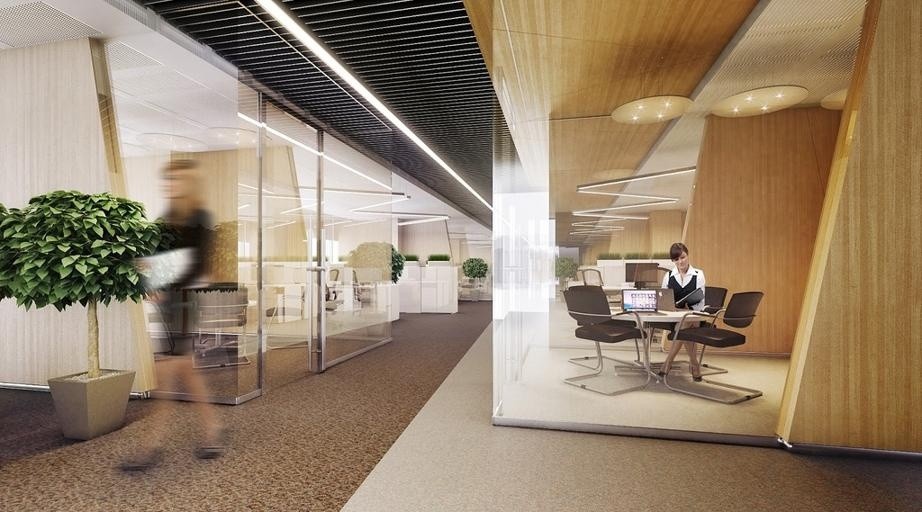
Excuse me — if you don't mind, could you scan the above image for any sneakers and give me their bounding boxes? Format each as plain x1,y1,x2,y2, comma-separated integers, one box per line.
117,421,238,472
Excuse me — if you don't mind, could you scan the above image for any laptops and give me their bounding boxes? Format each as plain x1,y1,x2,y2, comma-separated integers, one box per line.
646,287,690,313
621,288,668,317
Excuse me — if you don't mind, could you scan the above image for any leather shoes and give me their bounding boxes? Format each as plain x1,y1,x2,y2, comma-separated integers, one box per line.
688,365,703,383
658,365,673,378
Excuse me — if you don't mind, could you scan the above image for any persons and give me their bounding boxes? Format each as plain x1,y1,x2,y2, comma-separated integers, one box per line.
657,243,706,382
112,158,222,470
